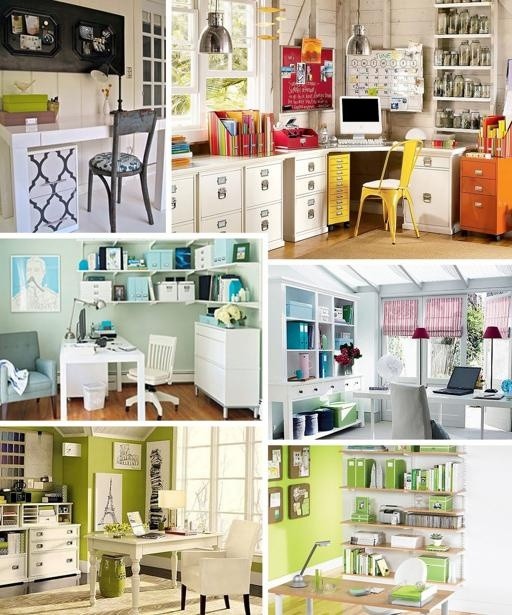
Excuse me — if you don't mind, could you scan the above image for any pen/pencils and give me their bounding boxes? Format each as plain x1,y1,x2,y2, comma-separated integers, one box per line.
484,394,494,397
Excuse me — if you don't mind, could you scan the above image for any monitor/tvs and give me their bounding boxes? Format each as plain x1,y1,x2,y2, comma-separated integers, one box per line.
75,308,90,343
338,94,383,140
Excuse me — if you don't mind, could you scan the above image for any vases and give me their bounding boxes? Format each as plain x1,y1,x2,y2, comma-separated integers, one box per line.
345,365,352,375
103,100,110,117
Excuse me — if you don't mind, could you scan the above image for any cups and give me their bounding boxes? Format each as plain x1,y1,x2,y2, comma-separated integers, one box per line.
329,136,338,148
391,516,398,525
318,128,329,144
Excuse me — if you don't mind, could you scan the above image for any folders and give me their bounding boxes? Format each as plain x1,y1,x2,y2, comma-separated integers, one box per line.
287,321,303,349
303,323,309,349
319,352,328,378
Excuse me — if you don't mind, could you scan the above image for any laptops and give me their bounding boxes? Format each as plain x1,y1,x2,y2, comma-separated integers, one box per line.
433,366,483,396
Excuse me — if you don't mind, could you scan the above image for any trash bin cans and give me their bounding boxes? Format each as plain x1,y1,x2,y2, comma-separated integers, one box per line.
82,382,105,411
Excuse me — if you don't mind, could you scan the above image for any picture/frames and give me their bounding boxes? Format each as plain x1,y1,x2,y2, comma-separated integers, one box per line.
233,242,250,262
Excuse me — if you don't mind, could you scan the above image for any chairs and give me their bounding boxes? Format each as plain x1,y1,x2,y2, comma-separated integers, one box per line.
180,519,262,615
353,148,461,235
87,108,158,233
389,382,432,439
0,330,58,420
158,490,186,532
354,139,425,245
125,334,180,420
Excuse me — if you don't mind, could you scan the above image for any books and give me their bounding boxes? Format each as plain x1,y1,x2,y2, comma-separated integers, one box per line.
403,461,464,492
405,516,464,529
172,137,192,168
345,549,389,575
0,532,25,554
478,119,507,138
220,114,273,152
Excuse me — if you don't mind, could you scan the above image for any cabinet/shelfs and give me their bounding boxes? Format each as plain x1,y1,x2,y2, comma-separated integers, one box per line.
78,240,191,302
433,1,498,133
172,150,351,259
0,524,81,585
459,156,512,241
195,321,261,420
20,501,74,526
269,277,365,440
339,446,464,579
187,239,262,309
0,503,20,526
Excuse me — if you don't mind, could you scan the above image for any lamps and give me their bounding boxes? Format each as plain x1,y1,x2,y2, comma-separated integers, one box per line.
289,540,331,588
62,442,81,457
412,328,430,385
199,0,234,54
64,297,107,339
345,0,370,57
91,60,128,114
483,326,502,393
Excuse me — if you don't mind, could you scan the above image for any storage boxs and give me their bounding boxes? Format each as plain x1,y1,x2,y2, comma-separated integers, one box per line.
286,300,313,320
0,111,56,126
144,250,174,269
154,281,177,302
177,280,195,302
3,95,48,113
320,402,358,428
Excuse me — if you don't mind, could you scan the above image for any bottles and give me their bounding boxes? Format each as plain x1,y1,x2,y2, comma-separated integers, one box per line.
437,8,489,35
435,108,480,129
434,39,491,66
434,72,490,98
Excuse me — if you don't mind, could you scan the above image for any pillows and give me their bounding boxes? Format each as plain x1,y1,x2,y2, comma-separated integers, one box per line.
432,420,451,439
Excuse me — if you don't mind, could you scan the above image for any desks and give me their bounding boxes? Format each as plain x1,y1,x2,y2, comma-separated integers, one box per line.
60,335,145,421
83,533,226,615
353,388,512,439
0,114,166,233
268,575,456,615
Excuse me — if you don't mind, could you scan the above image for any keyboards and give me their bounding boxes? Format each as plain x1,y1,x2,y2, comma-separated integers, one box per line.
339,140,385,147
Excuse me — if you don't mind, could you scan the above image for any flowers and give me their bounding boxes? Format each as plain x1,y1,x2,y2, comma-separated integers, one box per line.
214,304,248,329
102,83,112,100
333,342,362,367
